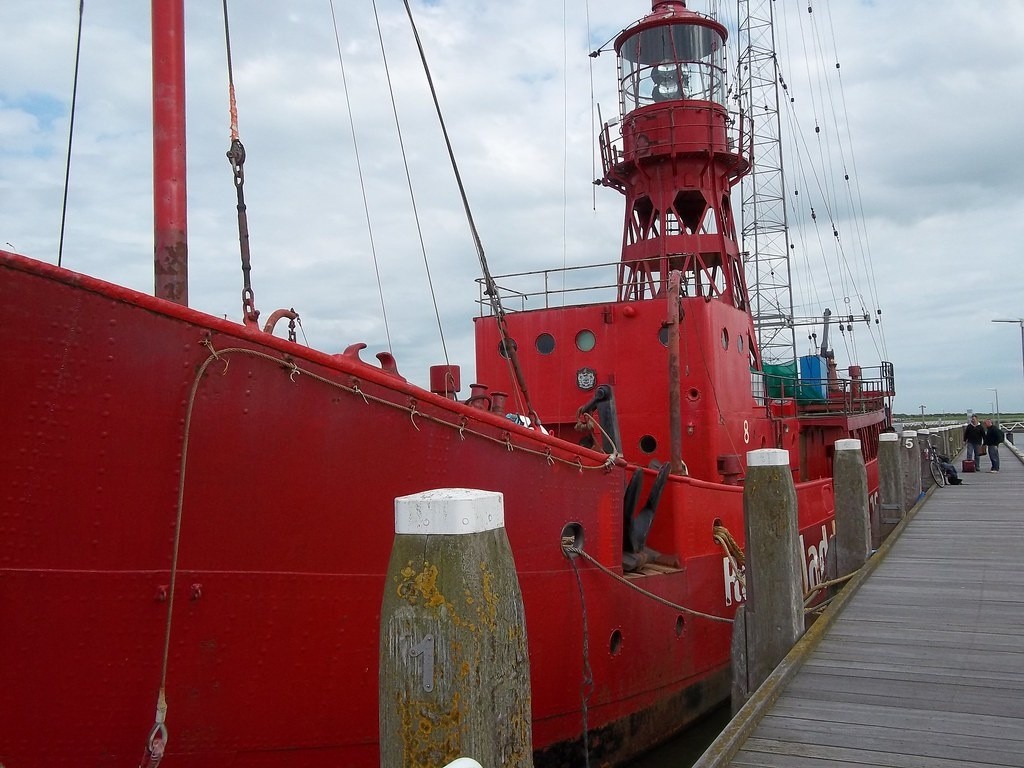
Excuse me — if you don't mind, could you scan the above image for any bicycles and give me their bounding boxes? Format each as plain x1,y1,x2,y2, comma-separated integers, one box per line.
918,433,950,488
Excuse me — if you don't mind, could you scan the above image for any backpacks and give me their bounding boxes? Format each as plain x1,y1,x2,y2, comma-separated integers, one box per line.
993,427,1005,443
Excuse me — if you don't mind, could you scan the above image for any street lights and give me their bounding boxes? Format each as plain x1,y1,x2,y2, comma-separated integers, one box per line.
992,389,1000,428
987,402,994,425
918,404,927,428
991,318,1024,378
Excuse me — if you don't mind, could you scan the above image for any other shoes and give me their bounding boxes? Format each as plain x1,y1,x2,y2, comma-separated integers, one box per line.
977,468,980,471
988,470,999,473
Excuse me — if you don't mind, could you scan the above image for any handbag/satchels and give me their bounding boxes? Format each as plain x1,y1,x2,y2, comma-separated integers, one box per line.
979,446,987,456
962,460,976,472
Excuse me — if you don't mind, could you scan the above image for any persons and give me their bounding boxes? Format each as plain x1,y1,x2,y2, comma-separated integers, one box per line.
983,418,1000,474
964,415,987,471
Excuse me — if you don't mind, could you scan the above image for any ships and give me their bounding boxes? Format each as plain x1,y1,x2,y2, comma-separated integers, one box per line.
1,0,897,767
